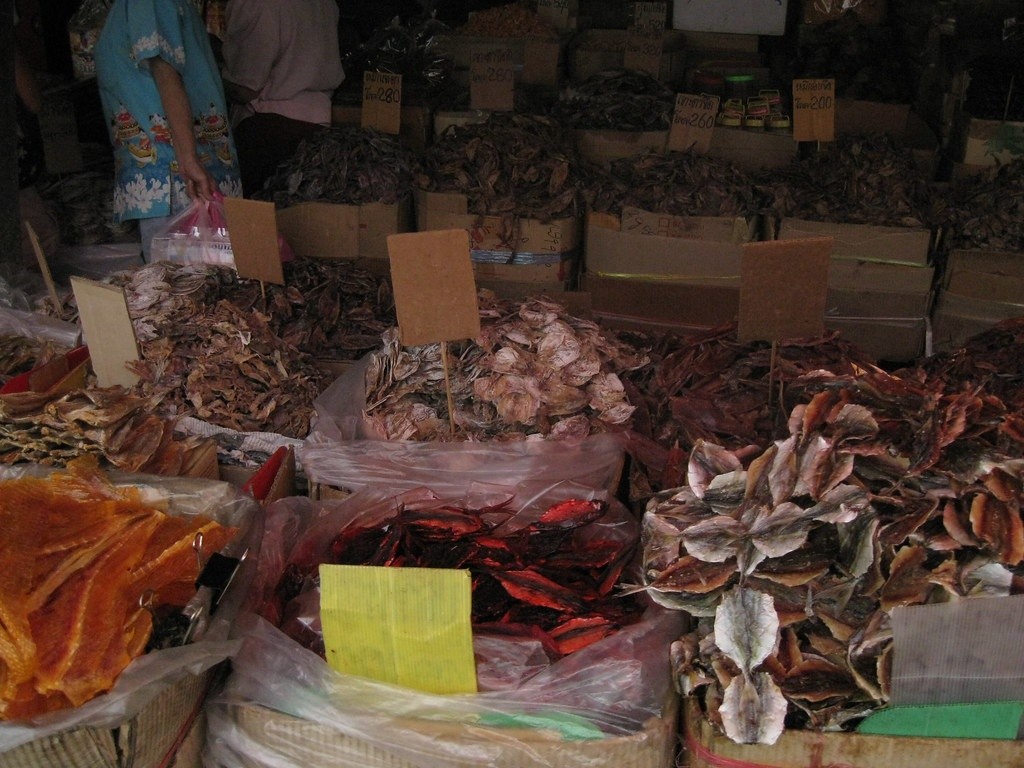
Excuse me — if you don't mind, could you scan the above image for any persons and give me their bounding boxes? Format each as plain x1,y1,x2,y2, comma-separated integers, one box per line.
218,0,345,199
1,0,244,278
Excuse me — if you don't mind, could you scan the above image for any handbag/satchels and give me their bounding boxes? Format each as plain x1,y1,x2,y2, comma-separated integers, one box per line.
151,191,296,270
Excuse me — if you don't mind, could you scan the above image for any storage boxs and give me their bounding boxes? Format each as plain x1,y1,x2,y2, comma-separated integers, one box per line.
1,0,1024,766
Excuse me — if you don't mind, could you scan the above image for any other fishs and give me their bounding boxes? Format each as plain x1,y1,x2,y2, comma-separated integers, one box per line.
0,0,1023,746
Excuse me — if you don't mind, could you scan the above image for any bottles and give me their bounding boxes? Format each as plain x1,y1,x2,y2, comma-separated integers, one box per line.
722,74,755,105
692,71,725,114
